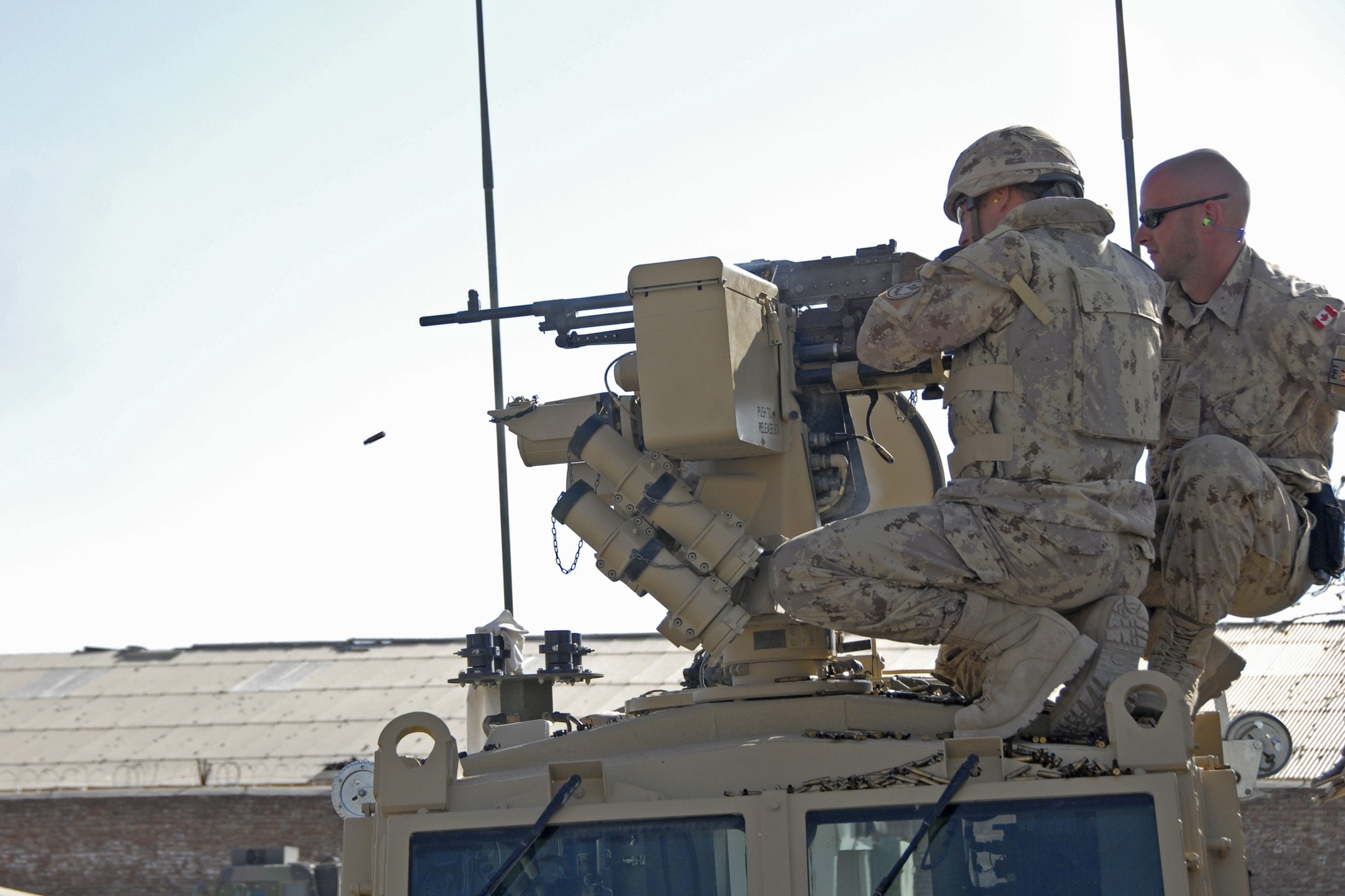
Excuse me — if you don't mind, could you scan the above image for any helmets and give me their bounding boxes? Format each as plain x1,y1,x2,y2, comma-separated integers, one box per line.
943,125,1084,225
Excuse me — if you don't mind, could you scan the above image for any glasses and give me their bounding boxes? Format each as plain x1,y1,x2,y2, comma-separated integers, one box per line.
1139,194,1228,229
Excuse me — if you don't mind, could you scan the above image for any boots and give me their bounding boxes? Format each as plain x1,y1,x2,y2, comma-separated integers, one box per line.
1050,595,1149,740
1134,606,1216,717
940,591,1098,739
1190,634,1247,723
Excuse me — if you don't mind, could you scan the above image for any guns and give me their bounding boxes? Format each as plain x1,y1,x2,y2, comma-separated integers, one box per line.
415,242,971,378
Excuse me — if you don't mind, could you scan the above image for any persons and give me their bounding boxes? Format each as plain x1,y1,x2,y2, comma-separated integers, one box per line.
1132,149,1345,717
767,127,1169,745
